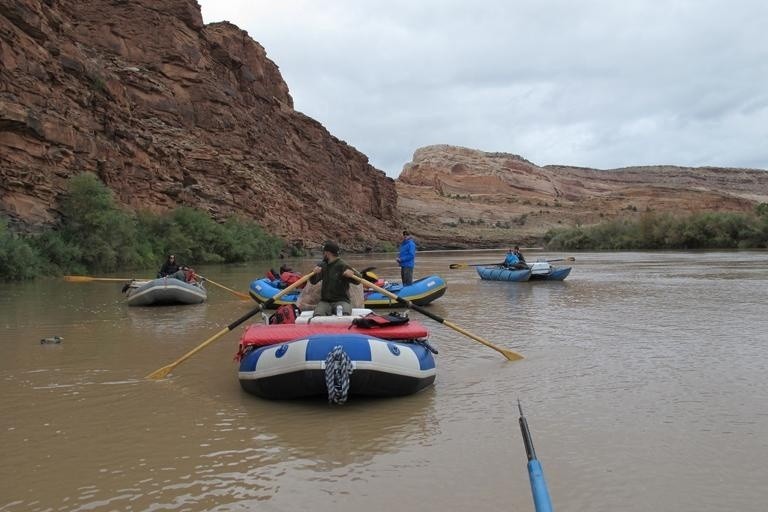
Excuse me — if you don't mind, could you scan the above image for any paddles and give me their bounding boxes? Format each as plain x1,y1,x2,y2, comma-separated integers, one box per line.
449,264,505,269
351,274,524,361
359,266,376,279
192,273,251,301
64,275,150,282
145,266,323,379
527,257,575,263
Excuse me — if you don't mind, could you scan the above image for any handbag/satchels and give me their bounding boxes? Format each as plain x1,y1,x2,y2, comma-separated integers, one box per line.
280,272,306,289
269,304,300,323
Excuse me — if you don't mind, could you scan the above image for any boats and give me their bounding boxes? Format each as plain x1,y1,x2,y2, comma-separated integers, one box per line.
476,265,572,281
248,276,448,307
127,279,207,306
238,333,437,400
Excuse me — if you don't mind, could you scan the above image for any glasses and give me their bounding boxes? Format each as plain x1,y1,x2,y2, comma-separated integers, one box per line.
403,234,410,236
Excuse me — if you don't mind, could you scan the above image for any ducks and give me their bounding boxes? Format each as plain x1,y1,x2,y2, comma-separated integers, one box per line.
40,336,64,344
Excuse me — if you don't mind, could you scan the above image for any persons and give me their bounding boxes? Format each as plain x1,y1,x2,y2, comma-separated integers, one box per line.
505,248,518,266
186,268,195,283
394,230,416,287
503,245,525,268
278,262,307,288
159,254,179,277
308,239,363,317
163,264,189,282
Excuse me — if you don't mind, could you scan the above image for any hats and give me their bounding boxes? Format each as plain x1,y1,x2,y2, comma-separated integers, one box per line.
320,243,338,254
